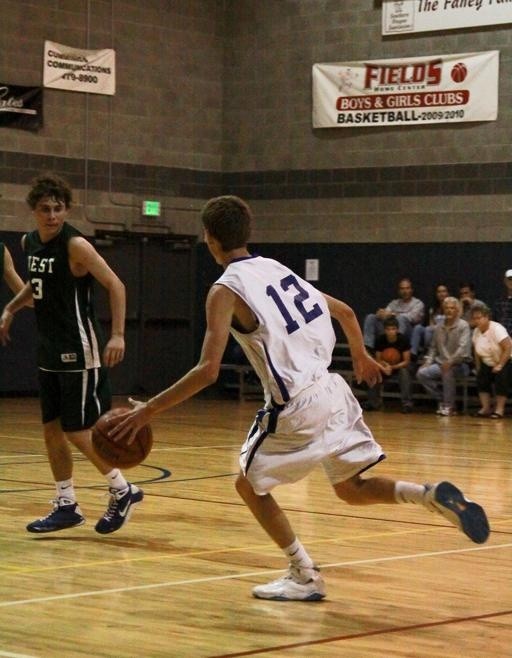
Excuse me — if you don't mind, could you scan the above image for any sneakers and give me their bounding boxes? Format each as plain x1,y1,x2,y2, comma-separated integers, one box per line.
93,480,145,535
421,478,490,544
25,494,85,532
252,568,327,603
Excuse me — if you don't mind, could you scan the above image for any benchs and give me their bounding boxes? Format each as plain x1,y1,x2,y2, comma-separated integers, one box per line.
231,334,512,413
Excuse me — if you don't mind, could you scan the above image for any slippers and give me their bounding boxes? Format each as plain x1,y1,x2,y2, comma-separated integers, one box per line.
470,411,490,418
489,412,505,419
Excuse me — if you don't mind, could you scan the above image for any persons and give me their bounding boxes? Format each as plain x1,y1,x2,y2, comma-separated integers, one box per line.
417,283,454,363
467,306,512,421
360,277,427,364
450,283,495,328
491,266,512,338
106,194,489,605
0,241,38,309
360,318,416,414
413,297,472,417
1,171,145,535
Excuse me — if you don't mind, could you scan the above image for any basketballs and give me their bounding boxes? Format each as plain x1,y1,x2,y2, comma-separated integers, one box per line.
91,407,153,469
382,348,400,365
451,62,468,82
